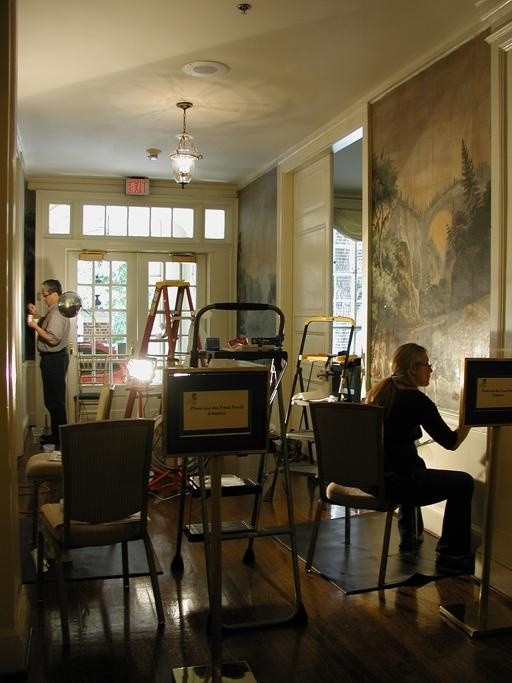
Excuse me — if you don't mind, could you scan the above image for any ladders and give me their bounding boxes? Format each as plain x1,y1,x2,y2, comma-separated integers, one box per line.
170,301,310,639
267,314,354,513
124,279,198,419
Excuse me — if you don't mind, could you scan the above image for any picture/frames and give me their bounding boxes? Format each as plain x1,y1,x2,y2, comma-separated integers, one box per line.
363,35,508,434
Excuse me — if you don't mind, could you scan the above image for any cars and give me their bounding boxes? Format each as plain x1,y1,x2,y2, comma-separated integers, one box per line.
78,342,127,385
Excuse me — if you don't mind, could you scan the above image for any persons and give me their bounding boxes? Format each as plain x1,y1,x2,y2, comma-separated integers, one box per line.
25,279,71,447
363,342,475,573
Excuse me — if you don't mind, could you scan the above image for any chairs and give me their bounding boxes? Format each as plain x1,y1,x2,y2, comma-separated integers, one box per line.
306,399,421,604
25,384,115,551
25,417,167,653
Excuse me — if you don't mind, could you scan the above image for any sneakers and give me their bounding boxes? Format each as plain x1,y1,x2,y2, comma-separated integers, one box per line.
397,535,423,550
434,550,475,562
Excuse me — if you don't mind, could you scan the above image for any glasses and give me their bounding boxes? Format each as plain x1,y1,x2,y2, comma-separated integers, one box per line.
416,360,431,367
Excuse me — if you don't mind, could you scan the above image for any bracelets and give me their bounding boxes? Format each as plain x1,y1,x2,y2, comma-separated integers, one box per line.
35,327,38,331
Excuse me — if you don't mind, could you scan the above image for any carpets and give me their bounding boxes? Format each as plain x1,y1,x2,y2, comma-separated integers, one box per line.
262,509,443,594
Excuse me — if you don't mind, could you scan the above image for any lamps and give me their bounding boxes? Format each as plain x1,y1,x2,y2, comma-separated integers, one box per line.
57,290,111,353
169,102,204,191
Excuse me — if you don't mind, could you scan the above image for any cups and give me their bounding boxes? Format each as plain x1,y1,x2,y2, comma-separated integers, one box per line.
28,315,34,322
43,444,55,460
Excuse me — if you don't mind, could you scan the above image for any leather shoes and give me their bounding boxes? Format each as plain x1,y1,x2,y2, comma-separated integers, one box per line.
41,433,55,438
40,437,58,443
